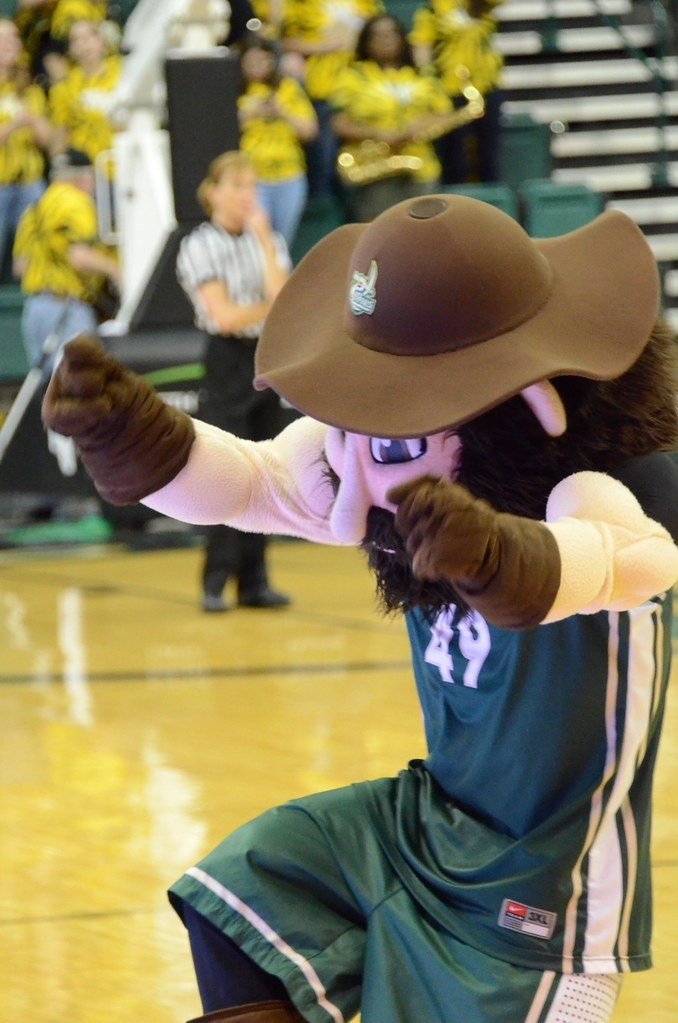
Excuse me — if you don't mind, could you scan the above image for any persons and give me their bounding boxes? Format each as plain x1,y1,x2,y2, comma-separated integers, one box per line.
0,0,128,480
175,153,292,612
234,0,505,248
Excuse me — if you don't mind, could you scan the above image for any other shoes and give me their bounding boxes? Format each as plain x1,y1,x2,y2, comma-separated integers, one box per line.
203,588,226,611
238,586,288,607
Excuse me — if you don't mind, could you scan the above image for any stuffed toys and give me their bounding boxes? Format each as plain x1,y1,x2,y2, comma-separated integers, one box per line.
42,192,678,1023
